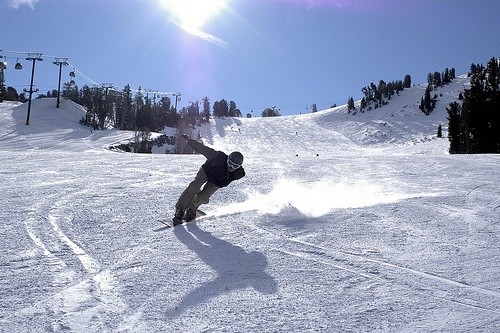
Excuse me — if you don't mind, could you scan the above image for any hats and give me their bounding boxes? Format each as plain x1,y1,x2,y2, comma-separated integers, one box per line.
229,152,244,165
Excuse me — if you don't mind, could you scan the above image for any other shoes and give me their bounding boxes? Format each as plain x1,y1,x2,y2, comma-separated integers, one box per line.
186,208,196,219
176,208,185,219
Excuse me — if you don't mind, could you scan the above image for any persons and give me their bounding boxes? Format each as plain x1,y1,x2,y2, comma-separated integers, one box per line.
172,134,246,226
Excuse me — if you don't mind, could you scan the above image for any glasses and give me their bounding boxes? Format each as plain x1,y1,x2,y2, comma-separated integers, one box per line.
227,159,240,169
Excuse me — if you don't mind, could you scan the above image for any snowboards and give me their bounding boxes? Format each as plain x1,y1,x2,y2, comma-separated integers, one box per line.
157,208,207,228
